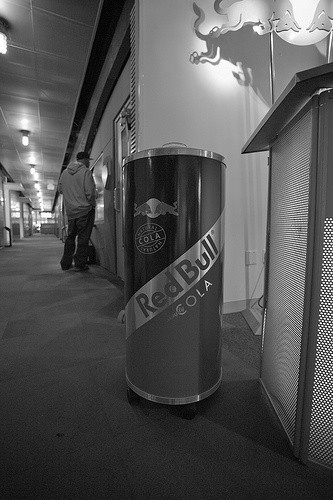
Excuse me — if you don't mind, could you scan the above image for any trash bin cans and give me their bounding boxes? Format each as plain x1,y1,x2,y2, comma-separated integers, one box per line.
121,142,227,407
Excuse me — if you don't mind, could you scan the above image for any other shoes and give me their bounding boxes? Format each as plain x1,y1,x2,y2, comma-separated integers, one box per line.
73,264,89,271
62,264,73,270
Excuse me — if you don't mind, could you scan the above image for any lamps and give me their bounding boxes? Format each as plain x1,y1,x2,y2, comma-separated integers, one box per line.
0,17,10,54
19,129,31,147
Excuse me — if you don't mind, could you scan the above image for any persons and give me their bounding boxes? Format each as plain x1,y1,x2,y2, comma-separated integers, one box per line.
58,152,96,272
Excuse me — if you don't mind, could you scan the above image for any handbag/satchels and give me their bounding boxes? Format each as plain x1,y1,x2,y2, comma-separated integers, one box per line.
86,239,96,264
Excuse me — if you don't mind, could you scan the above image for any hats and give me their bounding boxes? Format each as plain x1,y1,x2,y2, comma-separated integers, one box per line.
77,152,94,160
